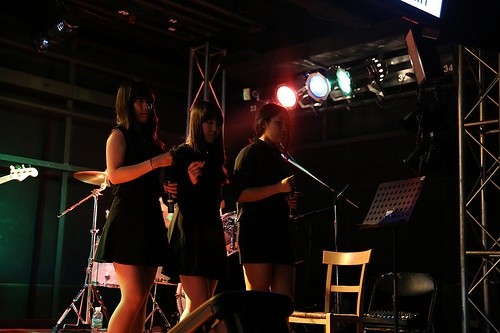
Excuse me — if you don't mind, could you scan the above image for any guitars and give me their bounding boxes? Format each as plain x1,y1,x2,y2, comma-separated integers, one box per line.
0,163,40,186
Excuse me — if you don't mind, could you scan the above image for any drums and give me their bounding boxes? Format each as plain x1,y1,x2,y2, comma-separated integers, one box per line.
155,267,178,285
90,235,120,288
219,212,241,254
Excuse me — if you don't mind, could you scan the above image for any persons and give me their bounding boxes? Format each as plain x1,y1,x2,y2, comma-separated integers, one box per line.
94,81,197,333
158,100,303,333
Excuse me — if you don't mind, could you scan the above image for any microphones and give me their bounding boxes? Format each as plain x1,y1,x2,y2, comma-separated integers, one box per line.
288,180,297,219
167,176,175,212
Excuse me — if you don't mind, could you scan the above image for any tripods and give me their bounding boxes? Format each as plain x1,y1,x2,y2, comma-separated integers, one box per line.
50,178,110,333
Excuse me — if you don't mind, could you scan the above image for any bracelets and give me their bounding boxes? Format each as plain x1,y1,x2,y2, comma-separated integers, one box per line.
149,158,155,169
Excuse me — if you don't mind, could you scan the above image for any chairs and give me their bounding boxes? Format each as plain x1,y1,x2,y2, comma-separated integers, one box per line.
287,249,372,333
363,272,437,333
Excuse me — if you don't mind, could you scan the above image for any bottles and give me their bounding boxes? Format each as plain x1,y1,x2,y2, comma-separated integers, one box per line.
90,307,103,333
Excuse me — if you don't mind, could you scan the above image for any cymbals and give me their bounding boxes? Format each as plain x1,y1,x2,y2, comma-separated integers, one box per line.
73,171,111,187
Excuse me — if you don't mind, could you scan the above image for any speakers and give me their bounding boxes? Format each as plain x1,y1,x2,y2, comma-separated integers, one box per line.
405,25,480,94
168,291,294,333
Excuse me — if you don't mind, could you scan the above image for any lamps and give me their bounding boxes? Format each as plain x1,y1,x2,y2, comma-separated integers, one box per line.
34,15,81,54
277,58,389,110
